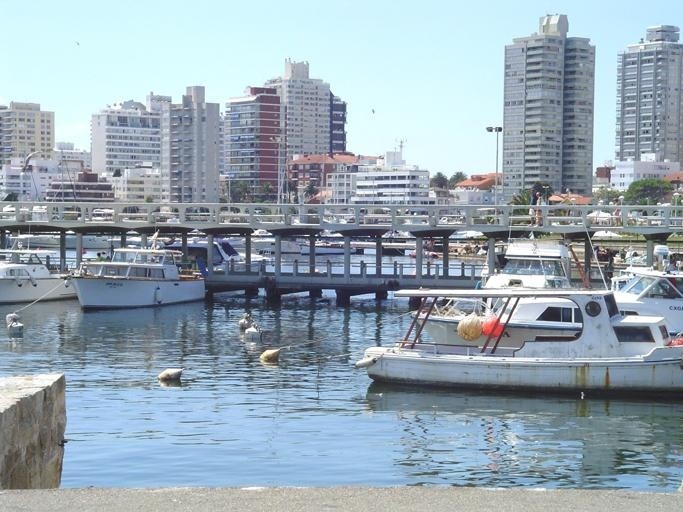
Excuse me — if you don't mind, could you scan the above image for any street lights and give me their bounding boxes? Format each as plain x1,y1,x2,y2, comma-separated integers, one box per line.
564,186,571,224
672,192,681,226
543,185,550,205
486,127,504,223
619,195,625,224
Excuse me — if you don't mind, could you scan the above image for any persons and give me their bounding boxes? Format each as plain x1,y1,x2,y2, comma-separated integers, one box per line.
612,198,622,227
533,191,543,226
528,192,535,227
